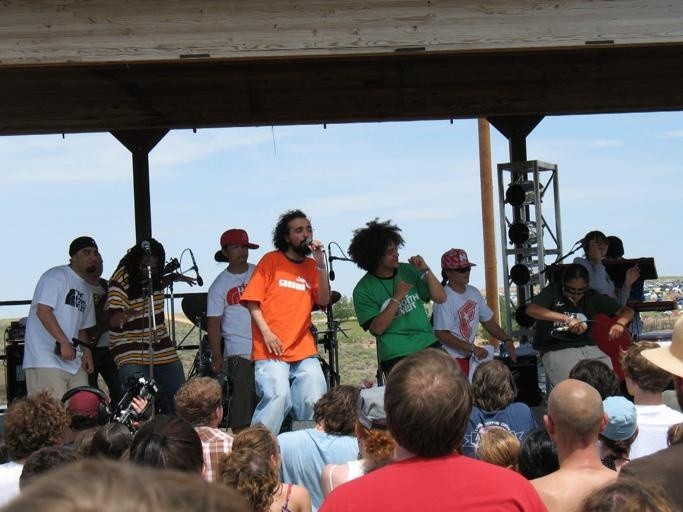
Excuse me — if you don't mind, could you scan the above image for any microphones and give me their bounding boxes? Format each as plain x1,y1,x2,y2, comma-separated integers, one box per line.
556,321,587,332
190,252,203,286
328,246,335,281
141,240,152,262
305,239,325,253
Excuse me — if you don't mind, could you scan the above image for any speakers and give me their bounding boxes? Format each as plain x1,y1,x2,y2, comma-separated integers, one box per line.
493,355,541,407
5,344,27,408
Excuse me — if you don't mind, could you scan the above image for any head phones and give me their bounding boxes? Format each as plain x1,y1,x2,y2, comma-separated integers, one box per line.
60,386,112,425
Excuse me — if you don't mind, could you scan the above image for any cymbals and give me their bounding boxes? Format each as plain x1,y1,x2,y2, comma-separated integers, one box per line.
313,291,341,310
181,293,209,332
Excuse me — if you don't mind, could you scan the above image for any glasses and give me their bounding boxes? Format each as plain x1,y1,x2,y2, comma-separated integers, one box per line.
452,267,471,273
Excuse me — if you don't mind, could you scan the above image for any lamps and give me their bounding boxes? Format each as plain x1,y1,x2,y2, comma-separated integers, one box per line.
496,159,562,352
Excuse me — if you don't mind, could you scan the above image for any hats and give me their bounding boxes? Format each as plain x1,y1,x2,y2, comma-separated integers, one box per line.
67,388,104,418
69,236,97,257
597,395,638,442
441,249,475,271
640,311,683,380
220,228,259,249
355,386,395,431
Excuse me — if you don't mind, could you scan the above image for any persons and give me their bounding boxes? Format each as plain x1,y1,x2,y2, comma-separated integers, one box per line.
0,314,683,511
239,209,332,434
434,231,645,399
23,229,259,432
347,214,453,380
